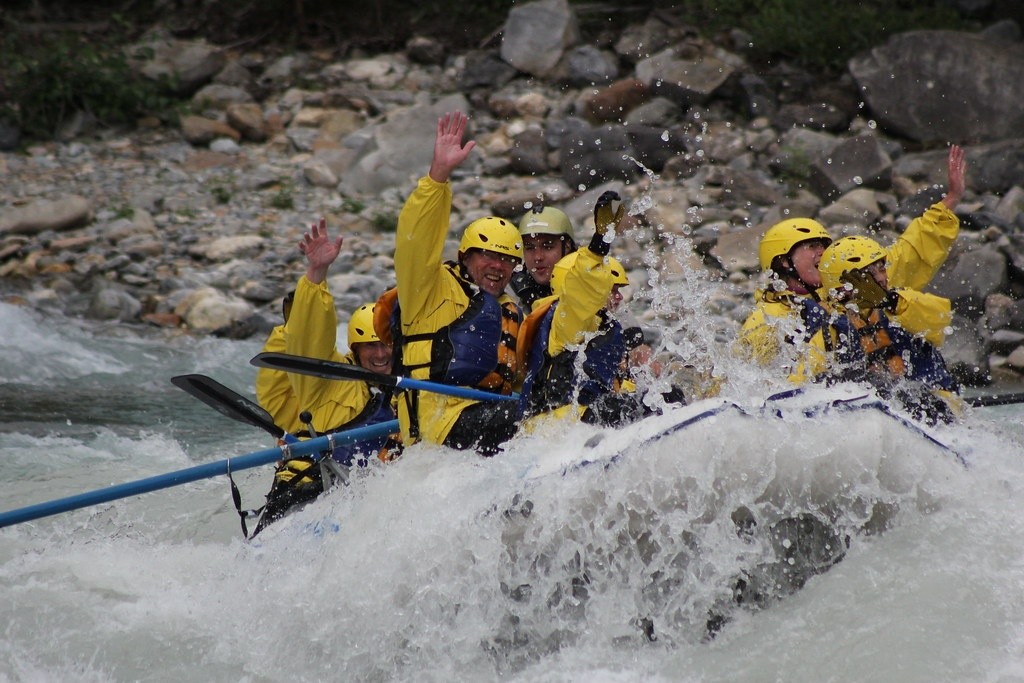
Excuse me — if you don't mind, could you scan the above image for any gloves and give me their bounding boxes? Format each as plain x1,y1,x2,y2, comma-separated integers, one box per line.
593,191,626,235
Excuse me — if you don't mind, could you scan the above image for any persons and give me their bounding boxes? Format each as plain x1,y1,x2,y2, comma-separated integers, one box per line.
528,190,628,420
255,289,326,532
505,199,578,415
393,117,526,458
583,326,685,429
285,217,393,481
705,144,968,421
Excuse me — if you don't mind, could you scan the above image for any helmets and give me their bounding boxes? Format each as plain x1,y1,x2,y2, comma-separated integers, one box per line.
550,251,630,295
518,206,576,251
459,216,523,265
759,217,833,273
347,303,379,348
818,236,888,292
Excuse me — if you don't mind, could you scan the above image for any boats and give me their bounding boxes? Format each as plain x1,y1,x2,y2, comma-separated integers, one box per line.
249,389,970,545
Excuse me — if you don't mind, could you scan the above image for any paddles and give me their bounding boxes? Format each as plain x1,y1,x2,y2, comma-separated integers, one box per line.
248,350,524,405
169,373,355,465
0,417,401,529
